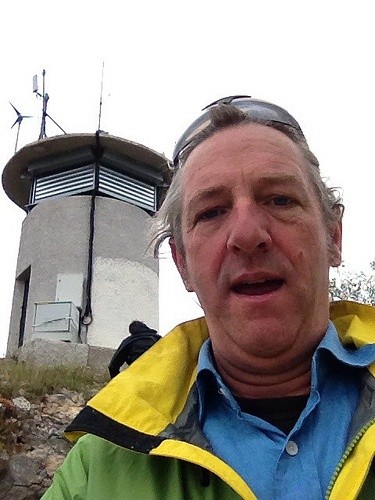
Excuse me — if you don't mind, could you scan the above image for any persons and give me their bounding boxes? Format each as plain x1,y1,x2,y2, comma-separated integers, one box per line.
40,99,375,500
108,321,163,380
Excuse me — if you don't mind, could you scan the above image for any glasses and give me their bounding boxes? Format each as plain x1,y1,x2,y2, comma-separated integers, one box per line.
172,93,298,165
